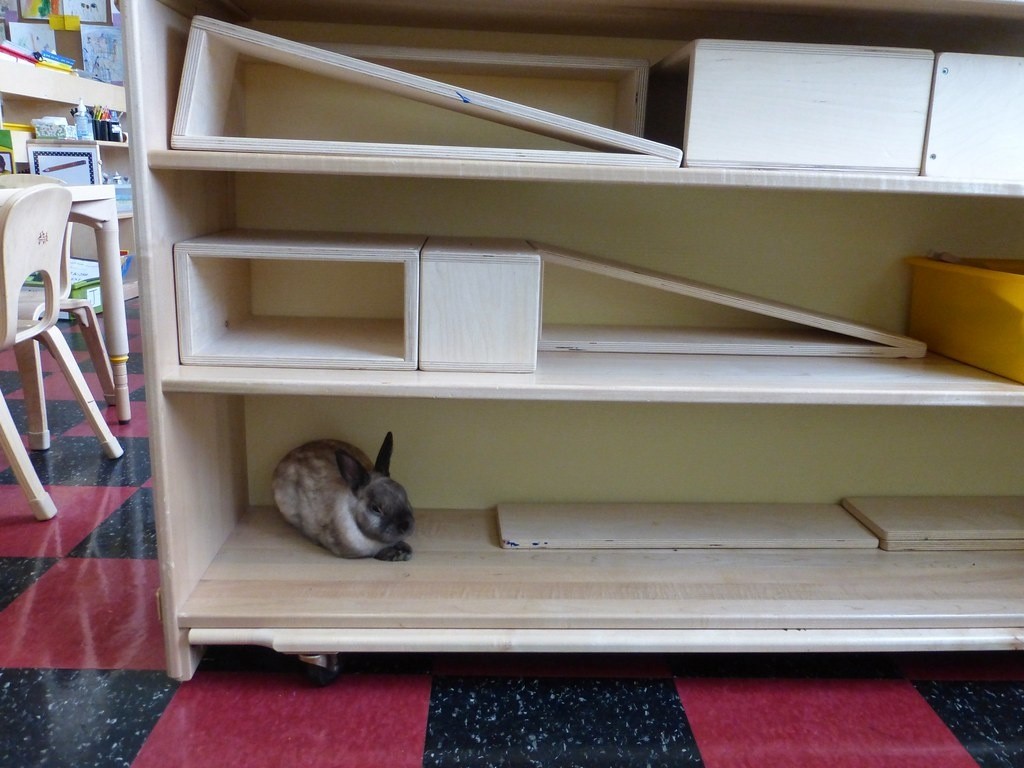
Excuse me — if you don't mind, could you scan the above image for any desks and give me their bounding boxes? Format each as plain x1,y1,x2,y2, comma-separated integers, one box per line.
0,183,132,424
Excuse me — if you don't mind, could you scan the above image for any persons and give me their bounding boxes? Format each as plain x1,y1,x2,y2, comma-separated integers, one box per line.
0,153,11,176
113,0,121,27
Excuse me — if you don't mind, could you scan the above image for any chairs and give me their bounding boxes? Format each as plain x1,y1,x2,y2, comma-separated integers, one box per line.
0,171,115,451
0,183,125,520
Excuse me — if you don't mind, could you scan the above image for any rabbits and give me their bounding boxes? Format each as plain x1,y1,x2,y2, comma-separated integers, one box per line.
267,431,415,562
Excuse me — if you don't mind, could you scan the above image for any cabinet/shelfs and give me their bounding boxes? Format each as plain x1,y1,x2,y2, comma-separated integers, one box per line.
119,1,1023,681
0,52,138,301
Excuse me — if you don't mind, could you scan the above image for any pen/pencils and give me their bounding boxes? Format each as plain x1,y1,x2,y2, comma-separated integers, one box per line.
69,104,124,122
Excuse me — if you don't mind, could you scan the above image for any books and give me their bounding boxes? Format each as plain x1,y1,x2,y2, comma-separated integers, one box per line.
0,38,76,74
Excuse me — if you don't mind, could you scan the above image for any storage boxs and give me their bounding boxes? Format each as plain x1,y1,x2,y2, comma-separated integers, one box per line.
908,256,1024,383
120,254,138,284
21,272,104,320
115,184,134,214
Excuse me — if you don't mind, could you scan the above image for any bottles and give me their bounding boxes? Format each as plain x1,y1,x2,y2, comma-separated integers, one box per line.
74,97,94,141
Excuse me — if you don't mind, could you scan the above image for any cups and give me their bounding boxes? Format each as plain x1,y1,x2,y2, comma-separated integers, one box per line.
91,117,124,142
